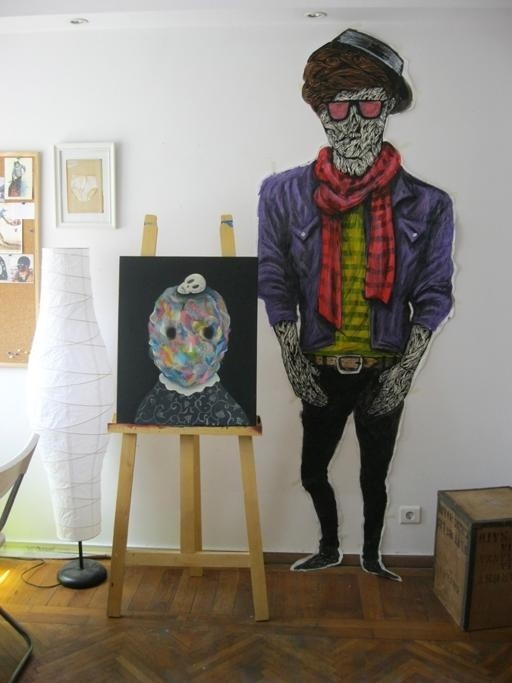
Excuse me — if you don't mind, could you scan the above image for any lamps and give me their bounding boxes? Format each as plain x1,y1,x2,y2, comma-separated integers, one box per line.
28,247,113,590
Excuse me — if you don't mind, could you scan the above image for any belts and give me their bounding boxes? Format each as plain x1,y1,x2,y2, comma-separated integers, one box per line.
301,350,404,376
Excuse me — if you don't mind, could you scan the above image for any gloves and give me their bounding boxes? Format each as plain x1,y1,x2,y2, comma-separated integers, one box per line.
272,320,329,409
366,323,432,418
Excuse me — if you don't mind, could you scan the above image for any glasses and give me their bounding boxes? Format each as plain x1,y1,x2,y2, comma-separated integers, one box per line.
320,95,395,122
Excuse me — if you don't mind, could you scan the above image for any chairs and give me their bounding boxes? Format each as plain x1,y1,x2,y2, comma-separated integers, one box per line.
0,433,39,683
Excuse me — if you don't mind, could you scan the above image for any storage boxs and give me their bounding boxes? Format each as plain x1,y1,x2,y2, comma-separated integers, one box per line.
430,485,512,633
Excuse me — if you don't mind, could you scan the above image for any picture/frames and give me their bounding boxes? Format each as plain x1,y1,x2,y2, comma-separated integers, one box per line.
0,151,42,368
52,141,115,231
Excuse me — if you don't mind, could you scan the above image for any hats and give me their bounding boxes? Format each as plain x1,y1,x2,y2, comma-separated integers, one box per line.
305,27,410,102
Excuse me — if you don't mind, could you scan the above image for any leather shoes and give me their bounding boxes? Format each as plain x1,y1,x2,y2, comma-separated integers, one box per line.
294,549,340,569
363,559,398,580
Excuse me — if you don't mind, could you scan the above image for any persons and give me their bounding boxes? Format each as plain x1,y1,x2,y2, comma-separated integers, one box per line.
13,256,34,283
257,26,454,584
